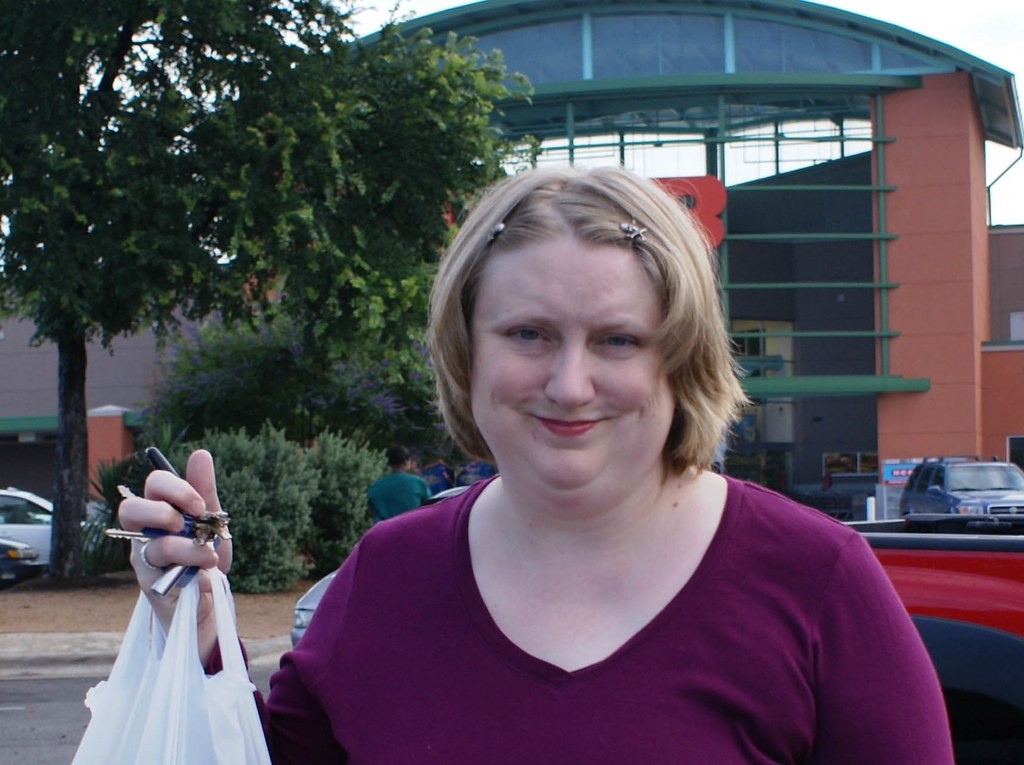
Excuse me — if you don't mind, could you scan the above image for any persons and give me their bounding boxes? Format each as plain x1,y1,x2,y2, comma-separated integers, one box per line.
364,445,431,523
119,164,954,765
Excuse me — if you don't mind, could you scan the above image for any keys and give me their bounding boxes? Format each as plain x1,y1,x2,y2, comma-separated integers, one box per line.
104,446,233,596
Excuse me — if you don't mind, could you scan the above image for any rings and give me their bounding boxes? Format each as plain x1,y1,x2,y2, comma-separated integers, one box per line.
140,542,158,571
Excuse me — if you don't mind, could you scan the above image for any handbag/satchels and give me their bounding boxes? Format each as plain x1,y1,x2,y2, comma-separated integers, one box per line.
71,562,271,765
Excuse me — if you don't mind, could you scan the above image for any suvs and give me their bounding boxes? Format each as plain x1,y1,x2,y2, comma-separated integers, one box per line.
899,455,1024,515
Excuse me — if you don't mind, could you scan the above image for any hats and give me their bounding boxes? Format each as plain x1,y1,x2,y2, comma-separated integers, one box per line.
385,443,412,465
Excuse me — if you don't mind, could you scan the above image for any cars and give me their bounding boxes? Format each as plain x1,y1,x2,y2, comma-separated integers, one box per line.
0,486,112,588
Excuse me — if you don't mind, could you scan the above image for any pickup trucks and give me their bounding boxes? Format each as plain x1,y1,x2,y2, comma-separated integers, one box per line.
811,515,1024,765
